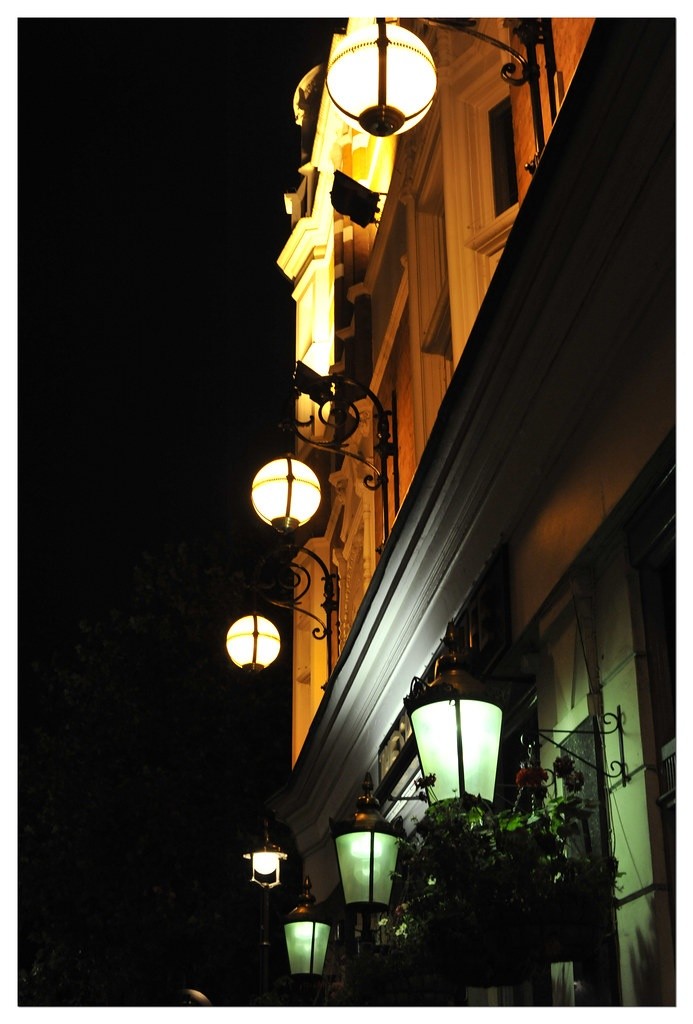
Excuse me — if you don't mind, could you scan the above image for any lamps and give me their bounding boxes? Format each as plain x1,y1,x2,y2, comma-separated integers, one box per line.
281,876,334,975
330,772,401,911
226,18,552,674
400,622,628,809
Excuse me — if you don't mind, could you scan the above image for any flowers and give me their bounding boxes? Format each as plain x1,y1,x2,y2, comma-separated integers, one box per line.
322,753,607,1007
